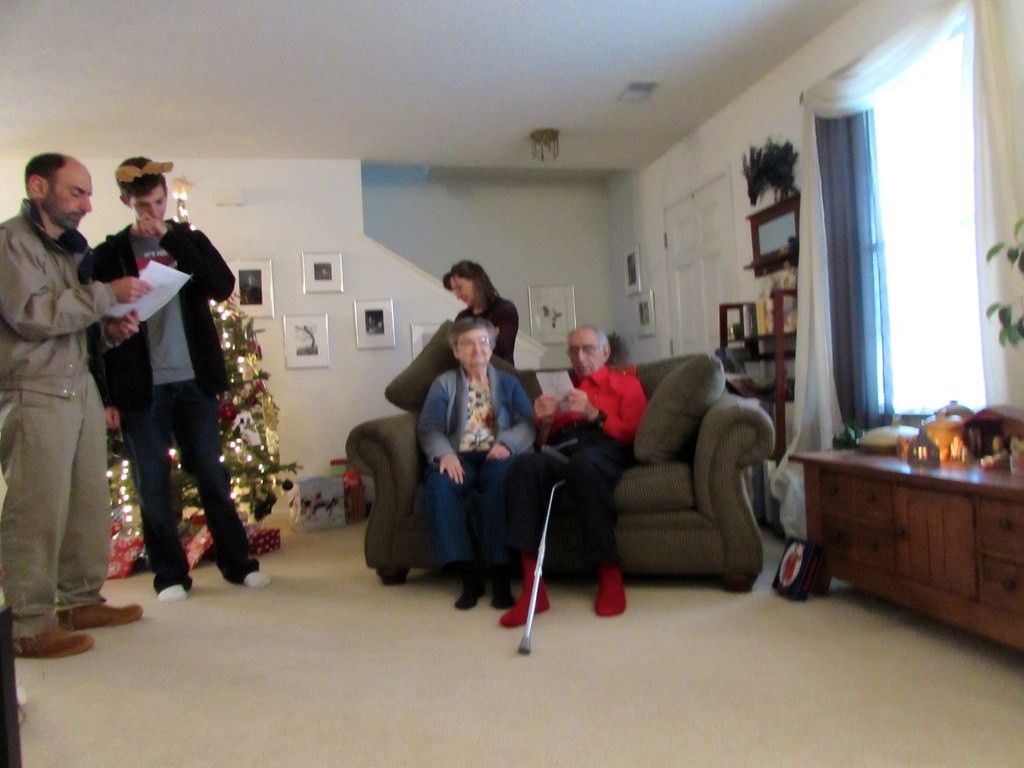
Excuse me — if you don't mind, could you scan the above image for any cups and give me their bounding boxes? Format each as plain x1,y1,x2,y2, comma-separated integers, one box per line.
742,302,758,338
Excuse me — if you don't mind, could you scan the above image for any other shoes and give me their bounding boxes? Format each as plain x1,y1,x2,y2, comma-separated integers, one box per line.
58,605,143,627
13,632,92,657
455,577,486,609
491,575,517,609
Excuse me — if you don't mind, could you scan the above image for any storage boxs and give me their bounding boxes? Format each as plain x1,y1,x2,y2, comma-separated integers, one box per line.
288,457,374,531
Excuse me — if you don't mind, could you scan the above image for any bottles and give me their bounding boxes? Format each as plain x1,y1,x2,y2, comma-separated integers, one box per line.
992,433,1024,476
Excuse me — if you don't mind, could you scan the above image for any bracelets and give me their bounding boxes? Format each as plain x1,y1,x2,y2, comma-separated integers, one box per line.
594,410,606,428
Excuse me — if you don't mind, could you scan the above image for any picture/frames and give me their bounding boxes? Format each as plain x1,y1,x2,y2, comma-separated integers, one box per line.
621,245,642,298
302,252,344,292
528,285,577,346
231,260,275,319
354,298,395,349
636,289,658,336
411,323,443,363
283,312,329,370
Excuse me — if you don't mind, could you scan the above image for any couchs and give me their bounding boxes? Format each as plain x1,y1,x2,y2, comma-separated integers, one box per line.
345,352,775,592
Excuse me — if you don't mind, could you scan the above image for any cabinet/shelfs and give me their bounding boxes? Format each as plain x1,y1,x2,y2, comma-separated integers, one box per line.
790,450,1024,650
719,289,796,460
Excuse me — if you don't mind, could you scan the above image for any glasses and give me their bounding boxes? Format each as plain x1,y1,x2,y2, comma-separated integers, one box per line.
456,336,491,349
568,346,602,354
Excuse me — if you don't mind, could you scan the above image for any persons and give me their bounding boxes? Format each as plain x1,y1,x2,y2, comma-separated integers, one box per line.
443,261,519,367
86,157,269,601
417,319,537,609
0,154,154,659
241,275,261,304
500,326,647,627
781,543,801,583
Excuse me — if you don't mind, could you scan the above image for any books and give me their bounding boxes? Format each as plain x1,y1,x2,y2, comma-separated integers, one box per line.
772,538,823,600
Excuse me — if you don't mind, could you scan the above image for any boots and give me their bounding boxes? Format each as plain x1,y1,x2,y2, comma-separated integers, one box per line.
500,559,550,627
596,569,626,615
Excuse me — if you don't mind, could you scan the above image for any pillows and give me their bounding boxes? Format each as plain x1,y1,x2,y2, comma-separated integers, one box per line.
633,352,725,461
383,319,515,412
